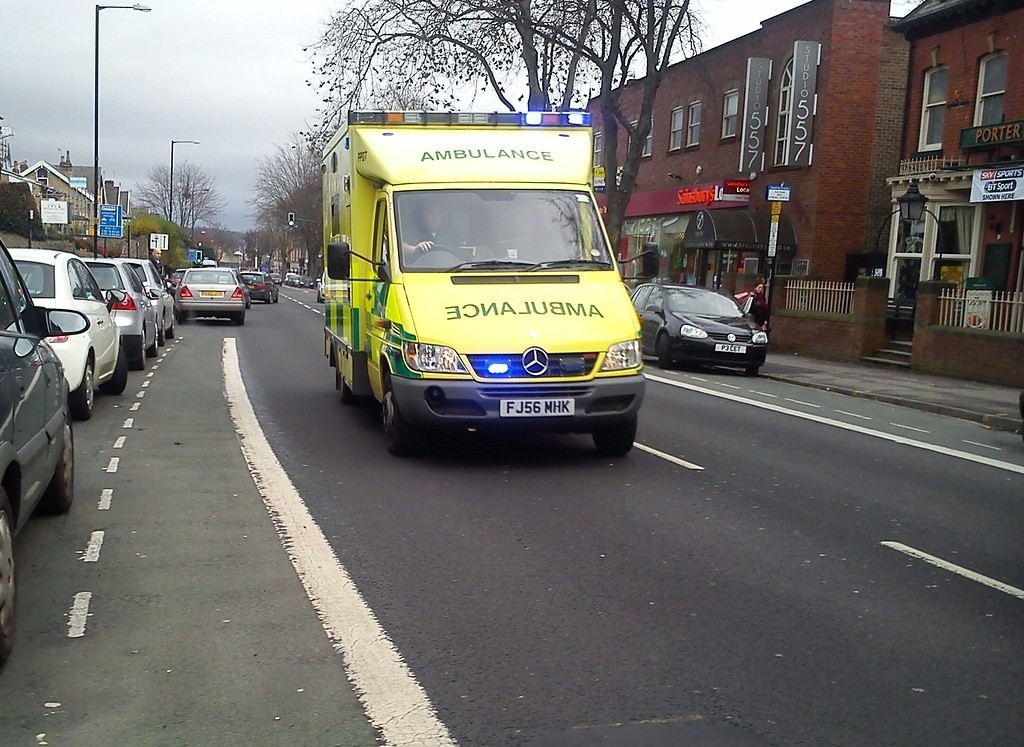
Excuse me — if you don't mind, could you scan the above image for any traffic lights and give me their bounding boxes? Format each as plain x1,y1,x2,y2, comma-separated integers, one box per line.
195,250,203,265
288,212,295,226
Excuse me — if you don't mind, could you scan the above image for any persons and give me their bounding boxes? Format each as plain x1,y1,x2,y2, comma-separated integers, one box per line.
148,248,171,288
401,198,476,264
743,280,771,331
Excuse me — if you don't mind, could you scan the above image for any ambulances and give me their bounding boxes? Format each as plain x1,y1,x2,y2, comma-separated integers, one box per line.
321,107,647,458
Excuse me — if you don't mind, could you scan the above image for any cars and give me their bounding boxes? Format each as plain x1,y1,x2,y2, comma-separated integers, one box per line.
7,246,130,419
67,256,163,370
0,237,75,665
221,267,252,309
94,256,178,346
270,271,314,288
629,280,770,376
315,271,326,303
175,267,247,325
238,270,280,304
166,269,187,298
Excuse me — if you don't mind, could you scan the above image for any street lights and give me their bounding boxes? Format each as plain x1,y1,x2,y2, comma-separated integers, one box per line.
93,4,153,261
192,189,210,242
169,140,200,221
